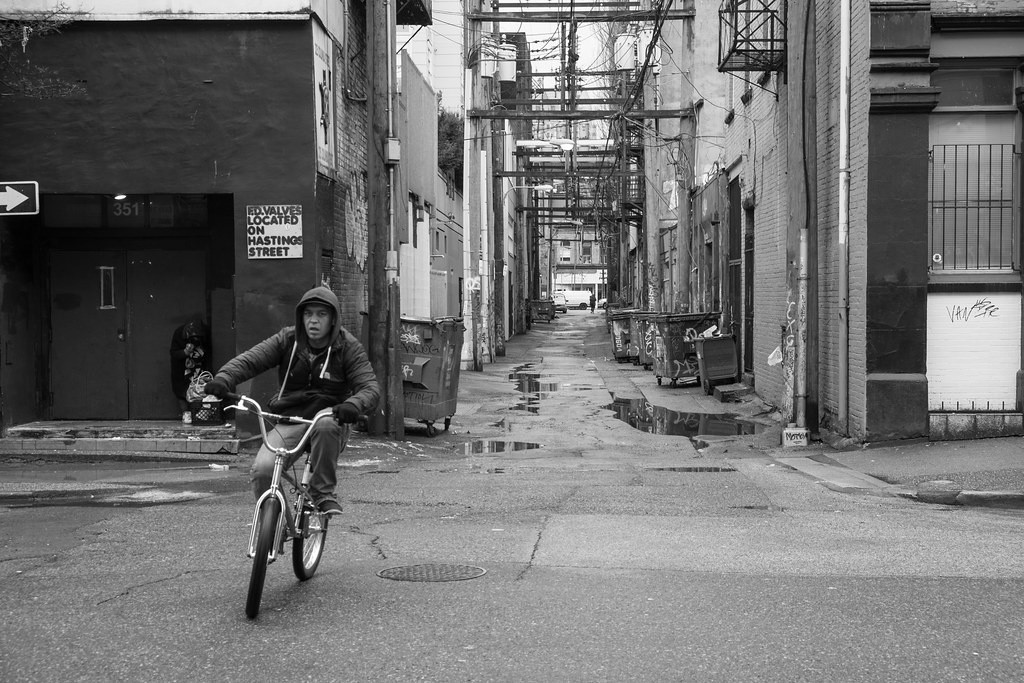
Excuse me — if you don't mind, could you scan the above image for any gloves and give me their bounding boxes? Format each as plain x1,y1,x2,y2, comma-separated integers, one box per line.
205,376,229,399
332,400,359,426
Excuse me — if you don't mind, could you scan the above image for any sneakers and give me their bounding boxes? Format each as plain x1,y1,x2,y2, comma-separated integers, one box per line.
182,411,192,424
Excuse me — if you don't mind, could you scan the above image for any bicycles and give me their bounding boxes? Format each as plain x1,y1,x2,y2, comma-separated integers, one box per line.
222,390,369,619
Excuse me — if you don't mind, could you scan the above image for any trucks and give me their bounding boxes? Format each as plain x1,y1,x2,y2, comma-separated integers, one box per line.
549,292,569,313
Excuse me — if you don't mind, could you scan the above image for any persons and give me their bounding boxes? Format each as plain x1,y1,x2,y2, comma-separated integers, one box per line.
170,318,211,423
590,294,596,314
204,287,380,515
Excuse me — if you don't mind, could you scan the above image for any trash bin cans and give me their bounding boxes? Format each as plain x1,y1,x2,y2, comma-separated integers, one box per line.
607,307,738,395
357,309,467,436
530,300,554,323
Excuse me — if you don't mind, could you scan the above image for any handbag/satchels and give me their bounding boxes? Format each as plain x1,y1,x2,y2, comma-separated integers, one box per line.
185,371,215,403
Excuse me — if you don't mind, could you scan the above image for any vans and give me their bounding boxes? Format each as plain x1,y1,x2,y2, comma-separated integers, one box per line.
555,290,592,310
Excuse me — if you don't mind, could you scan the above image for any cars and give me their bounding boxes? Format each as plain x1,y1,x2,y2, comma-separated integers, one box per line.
597,298,607,309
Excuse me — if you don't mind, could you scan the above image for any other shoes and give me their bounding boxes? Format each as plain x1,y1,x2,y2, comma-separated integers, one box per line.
318,503,343,515
